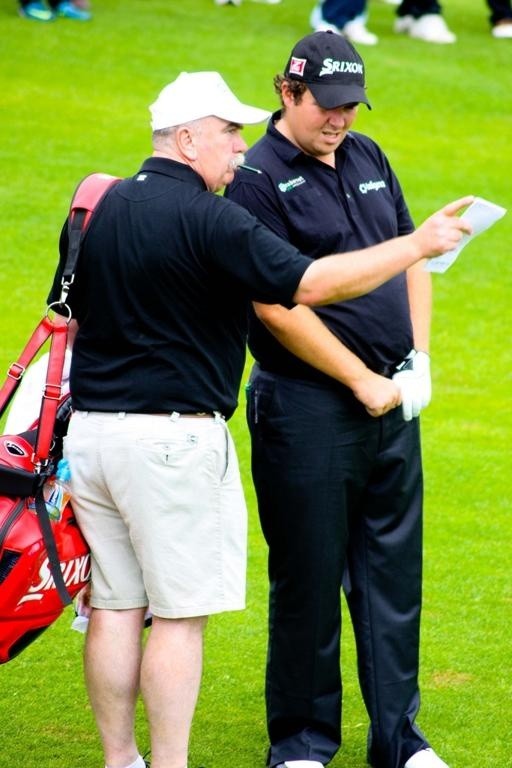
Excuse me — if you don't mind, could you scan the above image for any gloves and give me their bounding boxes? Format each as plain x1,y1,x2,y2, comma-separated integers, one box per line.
393,349,432,421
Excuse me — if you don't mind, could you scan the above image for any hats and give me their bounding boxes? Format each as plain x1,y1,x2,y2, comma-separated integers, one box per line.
148,69,273,130
283,32,373,112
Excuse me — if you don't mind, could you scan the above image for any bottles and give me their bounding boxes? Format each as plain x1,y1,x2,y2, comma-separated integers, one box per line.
32,460,74,523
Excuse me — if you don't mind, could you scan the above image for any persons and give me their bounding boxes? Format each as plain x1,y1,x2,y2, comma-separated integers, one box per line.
485,0,512,40
48,71,477,768
18,0,91,23
310,0,457,45
221,32,454,766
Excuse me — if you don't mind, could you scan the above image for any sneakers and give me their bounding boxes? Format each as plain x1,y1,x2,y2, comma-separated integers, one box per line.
309,7,379,45
404,747,450,768
394,14,457,44
18,2,90,23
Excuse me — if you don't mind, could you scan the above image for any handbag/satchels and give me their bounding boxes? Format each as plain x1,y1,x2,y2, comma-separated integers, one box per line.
0,314,103,663
